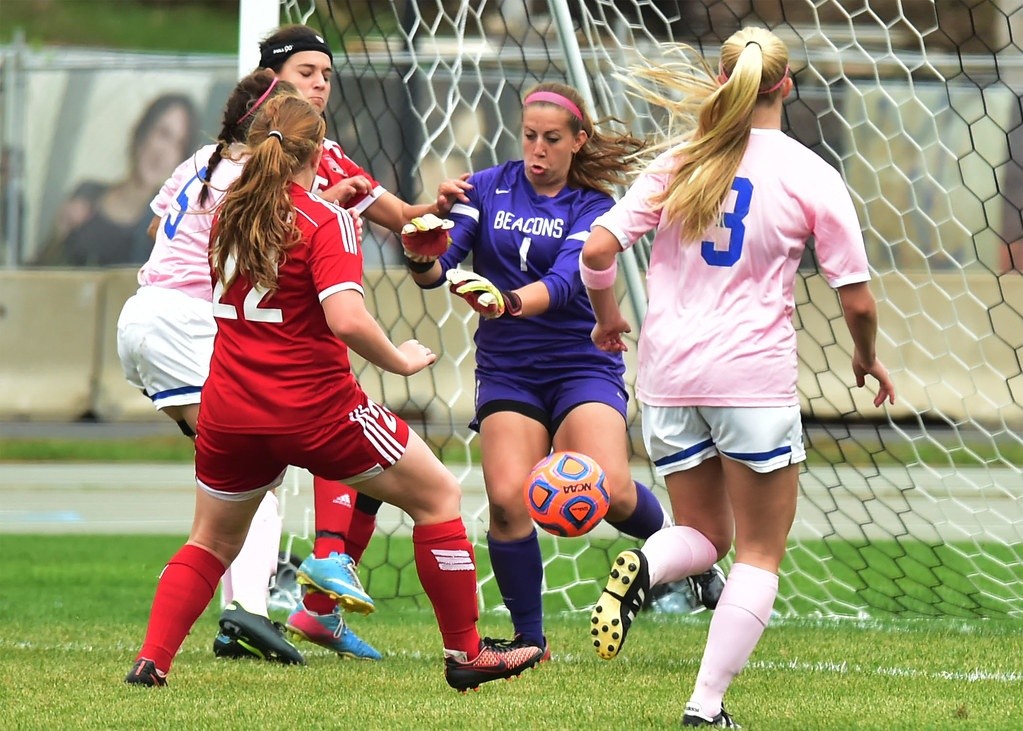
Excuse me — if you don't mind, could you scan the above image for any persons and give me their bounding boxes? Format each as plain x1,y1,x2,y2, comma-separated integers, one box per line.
400,83,726,662
580,27,894,727
117,68,304,665
124,95,544,689
39,94,197,268
260,24,474,659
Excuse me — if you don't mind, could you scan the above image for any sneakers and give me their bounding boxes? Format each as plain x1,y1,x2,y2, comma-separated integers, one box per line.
686,564,727,610
218,600,306,665
683,702,742,728
590,548,650,660
296,552,375,617
443,637,543,693
213,627,280,661
285,601,382,661
507,631,550,662
124,657,168,687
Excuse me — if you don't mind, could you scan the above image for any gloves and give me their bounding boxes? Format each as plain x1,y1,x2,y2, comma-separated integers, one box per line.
401,214,455,274
446,268,522,320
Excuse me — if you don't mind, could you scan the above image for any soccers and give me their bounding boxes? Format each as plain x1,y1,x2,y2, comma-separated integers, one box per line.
522,450,612,539
